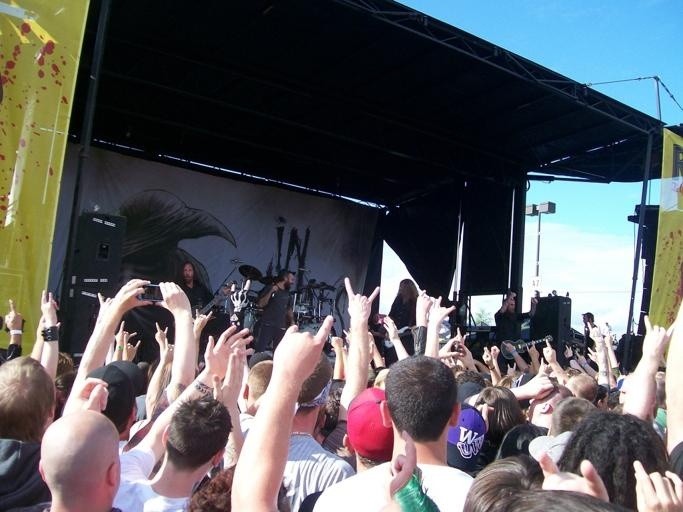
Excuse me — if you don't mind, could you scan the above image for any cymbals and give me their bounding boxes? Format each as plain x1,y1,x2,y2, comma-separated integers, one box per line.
260,277,284,284
309,284,334,290
238,265,263,282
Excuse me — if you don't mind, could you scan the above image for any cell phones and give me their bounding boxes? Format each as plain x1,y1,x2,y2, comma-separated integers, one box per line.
138,284,164,301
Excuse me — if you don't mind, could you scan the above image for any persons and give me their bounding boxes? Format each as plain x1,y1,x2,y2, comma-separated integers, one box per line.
231,315,334,512
243,360,273,409
563,322,626,413
62,278,195,454
106,321,167,383
57,352,78,408
447,402,486,479
111,325,243,512
32,316,45,364
465,375,573,431
313,356,474,512
540,452,610,503
665,297,683,482
367,279,566,389
623,316,675,419
474,387,527,437
311,278,380,472
0,300,22,365
634,461,683,512
389,430,441,512
175,263,220,318
321,336,345,438
188,465,290,512
254,270,295,353
144,338,200,417
9,410,123,512
557,413,670,509
282,351,354,512
0,357,57,512
300,389,394,512
41,290,60,383
547,397,598,437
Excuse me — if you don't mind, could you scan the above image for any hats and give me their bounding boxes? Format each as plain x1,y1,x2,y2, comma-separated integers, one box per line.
88,360,147,422
347,387,394,459
529,430,571,462
447,403,487,467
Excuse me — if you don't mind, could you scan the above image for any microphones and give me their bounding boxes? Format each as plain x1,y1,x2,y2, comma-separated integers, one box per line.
231,259,246,264
296,267,310,273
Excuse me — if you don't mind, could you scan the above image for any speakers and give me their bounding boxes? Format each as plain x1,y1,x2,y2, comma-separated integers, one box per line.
529,296,571,365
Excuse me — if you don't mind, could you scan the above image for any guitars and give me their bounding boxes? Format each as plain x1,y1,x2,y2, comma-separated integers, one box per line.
500,335,553,360
192,285,230,319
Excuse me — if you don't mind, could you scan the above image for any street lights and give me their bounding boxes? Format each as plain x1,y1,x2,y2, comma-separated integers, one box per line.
524,202,555,275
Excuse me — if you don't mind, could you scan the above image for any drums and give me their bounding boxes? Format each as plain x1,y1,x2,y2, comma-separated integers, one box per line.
248,320,258,336
291,303,309,314
303,323,336,355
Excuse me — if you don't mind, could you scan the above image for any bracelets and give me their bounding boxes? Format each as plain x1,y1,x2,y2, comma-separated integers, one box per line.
9,330,22,336
41,322,61,341
116,346,124,350
